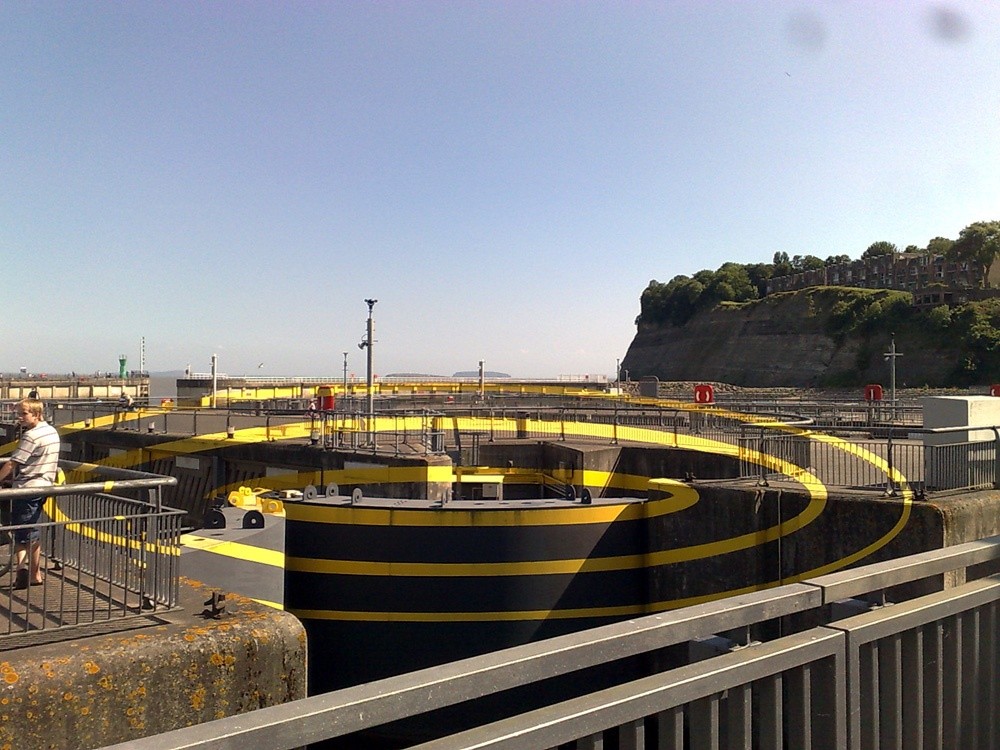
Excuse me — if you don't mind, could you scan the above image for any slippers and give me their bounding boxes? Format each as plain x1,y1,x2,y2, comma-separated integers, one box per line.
12,581,43,587
15,568,29,590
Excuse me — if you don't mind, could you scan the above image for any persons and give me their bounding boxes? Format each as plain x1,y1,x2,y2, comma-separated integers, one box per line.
0,400,60,591
306,399,319,417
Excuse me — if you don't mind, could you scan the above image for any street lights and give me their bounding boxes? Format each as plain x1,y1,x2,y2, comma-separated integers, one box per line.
357,298,379,451
